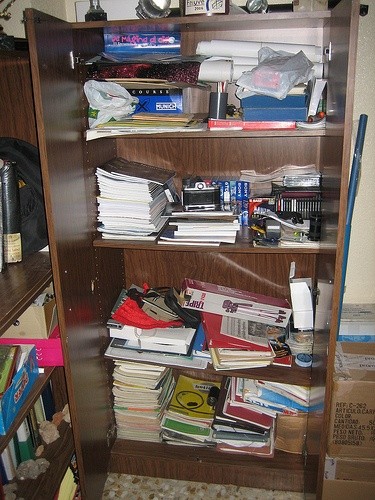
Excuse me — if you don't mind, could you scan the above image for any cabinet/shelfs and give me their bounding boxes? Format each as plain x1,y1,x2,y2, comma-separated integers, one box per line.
24,0,359,500
0,251,74,500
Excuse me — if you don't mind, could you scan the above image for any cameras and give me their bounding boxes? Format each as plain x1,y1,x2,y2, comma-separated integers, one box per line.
180,177,223,212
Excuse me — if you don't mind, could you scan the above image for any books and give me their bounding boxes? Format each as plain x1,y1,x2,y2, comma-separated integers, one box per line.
0,377,81,500
95,158,308,458
0,159,22,275
208,119,296,130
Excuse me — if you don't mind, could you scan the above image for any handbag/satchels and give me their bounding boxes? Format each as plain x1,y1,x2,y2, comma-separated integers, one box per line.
82,80,139,129
129,285,201,328
235,47,315,100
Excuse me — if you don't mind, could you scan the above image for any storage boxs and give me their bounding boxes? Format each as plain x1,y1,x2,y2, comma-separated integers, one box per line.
180,278,293,328
0,298,65,436
129,89,184,114
102,28,181,56
320,303,375,500
240,76,328,122
273,413,307,456
314,278,333,330
289,260,314,331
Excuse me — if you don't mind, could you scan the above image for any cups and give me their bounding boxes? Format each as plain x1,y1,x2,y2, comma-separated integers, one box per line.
208,92,228,118
